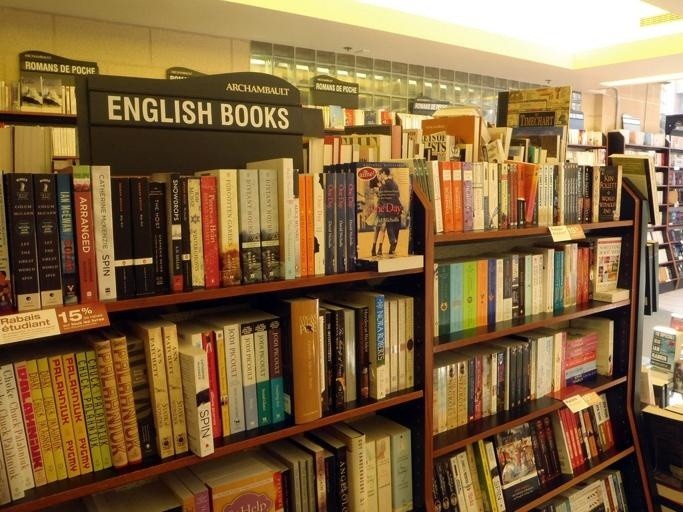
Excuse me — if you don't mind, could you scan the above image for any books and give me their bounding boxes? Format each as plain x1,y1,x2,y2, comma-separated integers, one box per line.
637,131,683,512
386,85,637,234
430,235,629,347
435,316,614,437
434,392,634,512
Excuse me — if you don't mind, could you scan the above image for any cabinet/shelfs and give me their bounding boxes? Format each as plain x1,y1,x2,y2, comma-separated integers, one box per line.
0,170,436,511
624,142,683,294
428,180,654,512
